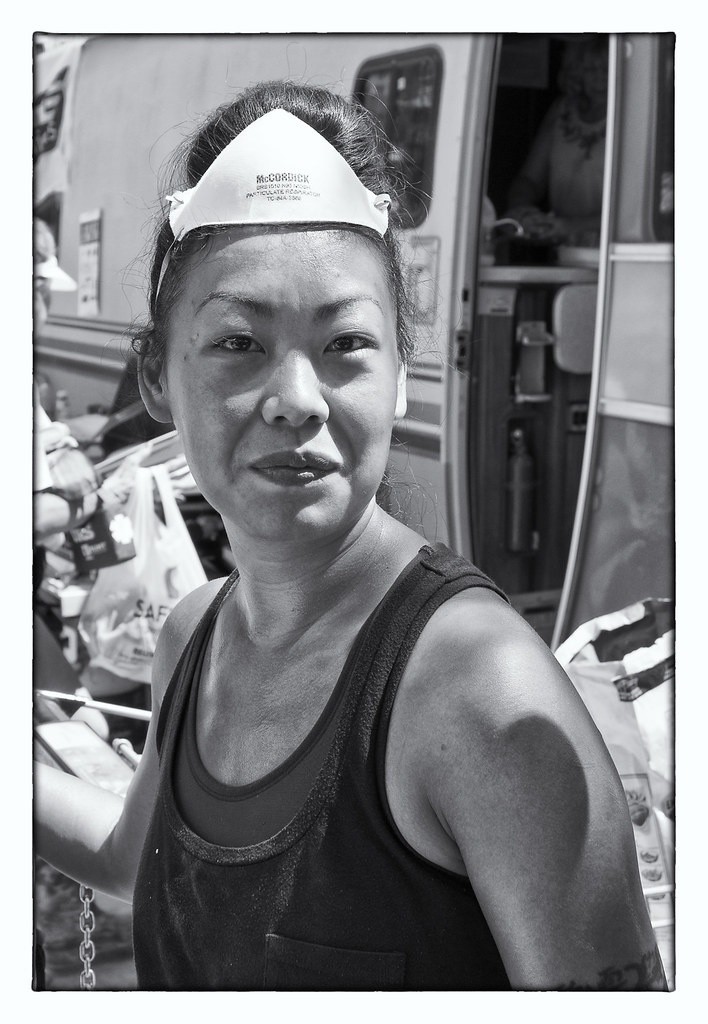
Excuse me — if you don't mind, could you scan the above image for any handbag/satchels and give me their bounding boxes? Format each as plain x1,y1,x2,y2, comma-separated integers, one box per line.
78,464,211,683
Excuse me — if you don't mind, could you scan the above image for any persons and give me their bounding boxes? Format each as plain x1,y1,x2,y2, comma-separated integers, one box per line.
509,38,609,244
33,83,668,989
